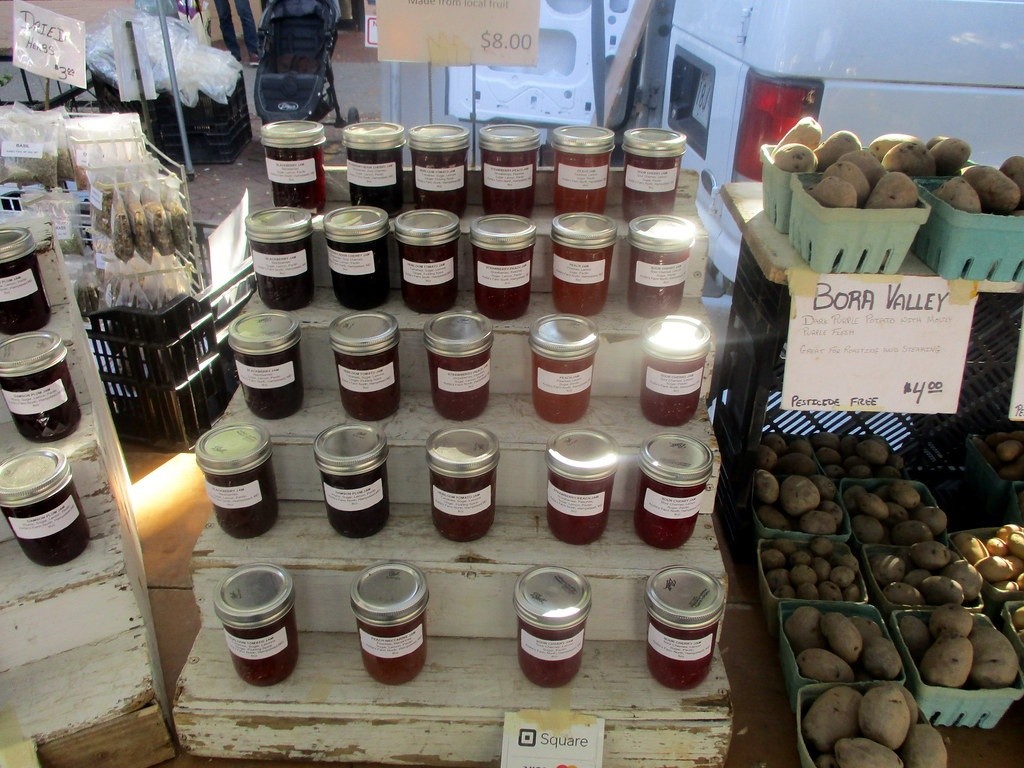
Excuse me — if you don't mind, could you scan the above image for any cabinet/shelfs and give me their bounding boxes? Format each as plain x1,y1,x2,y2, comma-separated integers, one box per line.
712,182,1023,521
0,219,171,768
172,166,735,768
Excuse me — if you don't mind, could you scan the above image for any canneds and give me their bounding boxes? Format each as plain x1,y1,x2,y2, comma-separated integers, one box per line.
198,119,724,690
1,227,91,568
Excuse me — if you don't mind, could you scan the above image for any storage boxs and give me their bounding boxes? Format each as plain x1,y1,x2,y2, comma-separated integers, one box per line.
79,221,254,439
751,144,1024,768
166,73,252,164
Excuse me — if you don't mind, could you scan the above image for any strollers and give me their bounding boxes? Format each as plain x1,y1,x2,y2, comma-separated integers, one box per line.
254,0,360,130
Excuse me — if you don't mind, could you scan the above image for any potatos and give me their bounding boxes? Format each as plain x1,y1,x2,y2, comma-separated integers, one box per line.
751,427,1024,768
772,115,1024,221
